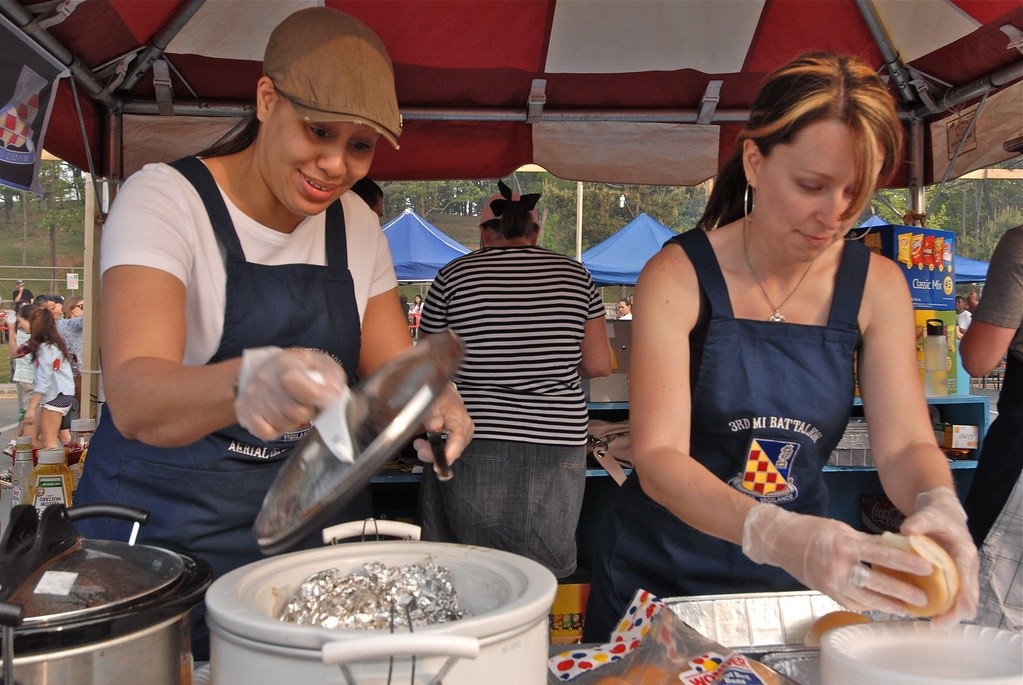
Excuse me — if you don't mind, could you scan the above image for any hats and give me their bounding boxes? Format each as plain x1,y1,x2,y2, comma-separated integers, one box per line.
55,295,65,303
262,7,404,151
479,180,540,249
16,280,25,285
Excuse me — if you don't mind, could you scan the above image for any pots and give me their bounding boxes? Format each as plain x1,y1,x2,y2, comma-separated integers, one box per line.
0,504,215,685
205,518,558,685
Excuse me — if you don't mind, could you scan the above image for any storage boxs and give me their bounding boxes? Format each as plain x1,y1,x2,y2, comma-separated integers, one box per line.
932,422,980,451
549,583,594,646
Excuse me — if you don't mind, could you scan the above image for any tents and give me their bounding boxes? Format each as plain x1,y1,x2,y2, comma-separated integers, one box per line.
0,0,1023,450
378,209,472,283
859,214,989,283
573,212,678,300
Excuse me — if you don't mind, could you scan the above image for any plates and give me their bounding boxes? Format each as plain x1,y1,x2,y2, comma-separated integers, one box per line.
818,620,1023,685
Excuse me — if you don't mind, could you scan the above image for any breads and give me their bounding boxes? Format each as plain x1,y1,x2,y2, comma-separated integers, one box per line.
804,611,871,647
594,654,783,685
871,531,959,618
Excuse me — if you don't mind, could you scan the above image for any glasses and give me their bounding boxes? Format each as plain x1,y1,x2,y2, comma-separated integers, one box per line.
75,303,83,309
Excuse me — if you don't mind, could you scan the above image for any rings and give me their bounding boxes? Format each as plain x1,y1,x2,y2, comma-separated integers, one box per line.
851,564,869,588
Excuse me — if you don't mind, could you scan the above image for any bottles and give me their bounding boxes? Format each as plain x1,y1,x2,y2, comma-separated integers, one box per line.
924,319,948,398
10,436,35,509
7,310,16,324
27,447,75,520
67,418,96,504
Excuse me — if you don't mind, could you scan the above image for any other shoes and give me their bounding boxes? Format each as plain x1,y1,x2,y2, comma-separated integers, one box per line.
2,443,15,457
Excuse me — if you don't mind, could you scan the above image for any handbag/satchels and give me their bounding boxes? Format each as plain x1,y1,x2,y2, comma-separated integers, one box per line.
586,418,634,487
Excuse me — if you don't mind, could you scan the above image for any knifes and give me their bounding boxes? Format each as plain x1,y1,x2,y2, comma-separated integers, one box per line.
426,430,449,477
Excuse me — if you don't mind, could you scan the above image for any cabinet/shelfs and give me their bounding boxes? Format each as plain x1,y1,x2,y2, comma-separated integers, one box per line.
364,395,989,530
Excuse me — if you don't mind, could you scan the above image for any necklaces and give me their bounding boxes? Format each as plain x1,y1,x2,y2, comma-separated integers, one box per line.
741,218,813,322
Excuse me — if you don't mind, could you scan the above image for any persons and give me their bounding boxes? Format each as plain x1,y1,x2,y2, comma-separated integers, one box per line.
414,179,611,580
410,294,425,337
69,6,473,662
400,295,410,325
0,281,85,456
615,295,634,321
352,177,383,221
953,296,972,339
579,52,978,630
961,224,1023,633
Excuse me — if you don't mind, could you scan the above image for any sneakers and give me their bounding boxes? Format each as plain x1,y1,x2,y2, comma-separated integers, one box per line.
0,468,12,487
